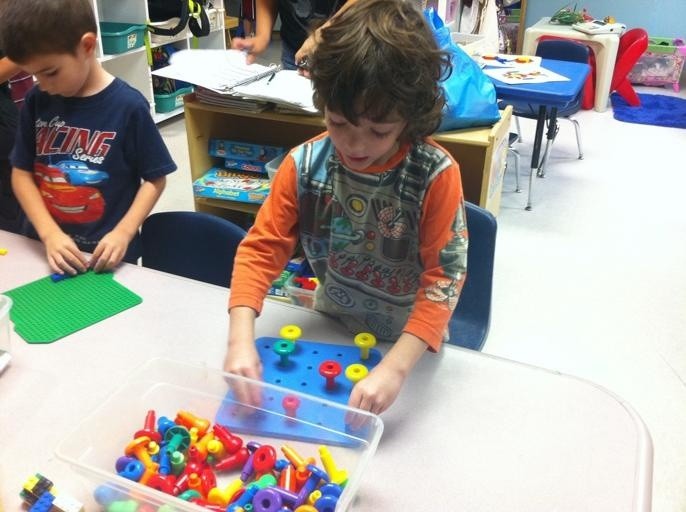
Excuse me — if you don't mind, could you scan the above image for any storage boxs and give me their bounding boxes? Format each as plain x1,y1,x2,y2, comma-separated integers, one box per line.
52,356,384,512
626,36,686,92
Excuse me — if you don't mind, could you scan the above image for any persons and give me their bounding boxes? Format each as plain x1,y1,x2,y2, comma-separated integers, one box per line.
0,46,26,235
0,0,177,277
222,0,469,433
231,0,357,80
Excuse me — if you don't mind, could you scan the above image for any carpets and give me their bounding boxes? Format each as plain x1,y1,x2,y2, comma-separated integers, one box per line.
610,91,685,129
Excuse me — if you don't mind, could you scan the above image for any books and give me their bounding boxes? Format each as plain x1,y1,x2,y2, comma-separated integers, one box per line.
150,49,324,118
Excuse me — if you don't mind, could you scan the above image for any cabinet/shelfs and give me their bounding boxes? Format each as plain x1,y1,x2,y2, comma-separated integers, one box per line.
89,0,226,124
181,93,513,304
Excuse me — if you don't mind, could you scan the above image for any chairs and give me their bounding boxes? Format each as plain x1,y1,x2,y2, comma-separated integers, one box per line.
437,201,498,352
580,28,647,109
496,39,590,178
122,209,247,289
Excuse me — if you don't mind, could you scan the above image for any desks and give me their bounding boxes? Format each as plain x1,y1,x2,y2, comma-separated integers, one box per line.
0,230,652,512
521,17,619,113
481,55,590,211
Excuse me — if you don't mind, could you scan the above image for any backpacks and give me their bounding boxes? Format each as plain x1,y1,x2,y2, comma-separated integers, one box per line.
143,1,209,37
